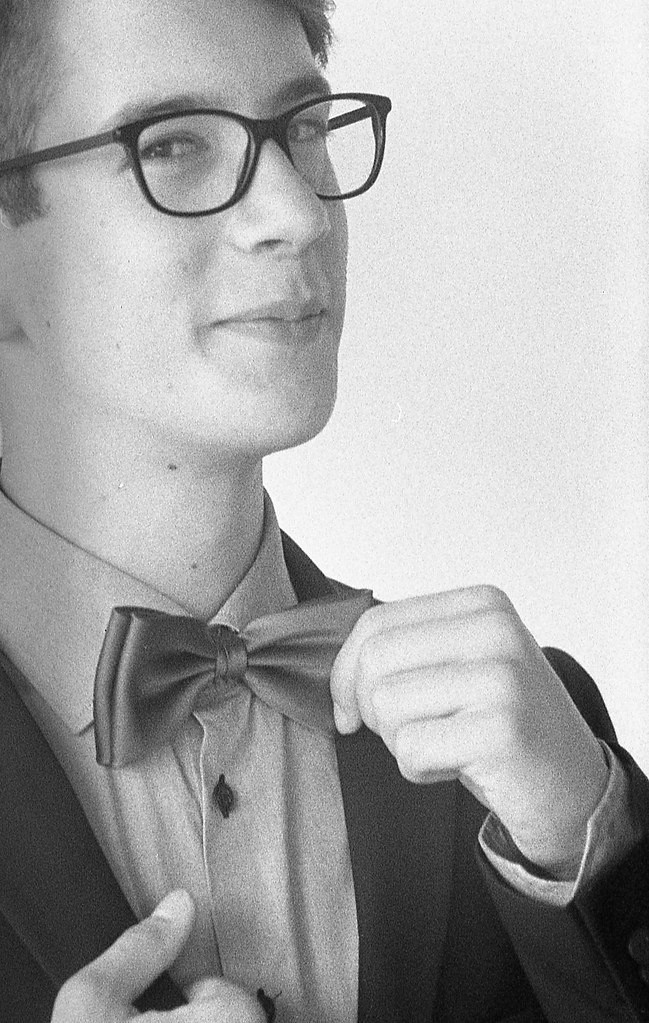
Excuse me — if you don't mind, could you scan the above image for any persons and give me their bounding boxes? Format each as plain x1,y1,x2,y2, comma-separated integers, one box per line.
0,1,649,1023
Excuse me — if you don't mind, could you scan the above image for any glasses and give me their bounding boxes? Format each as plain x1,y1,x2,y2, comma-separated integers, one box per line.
2,87,393,218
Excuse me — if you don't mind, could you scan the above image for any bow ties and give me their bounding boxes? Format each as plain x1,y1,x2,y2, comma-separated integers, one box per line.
92,590,373,768
